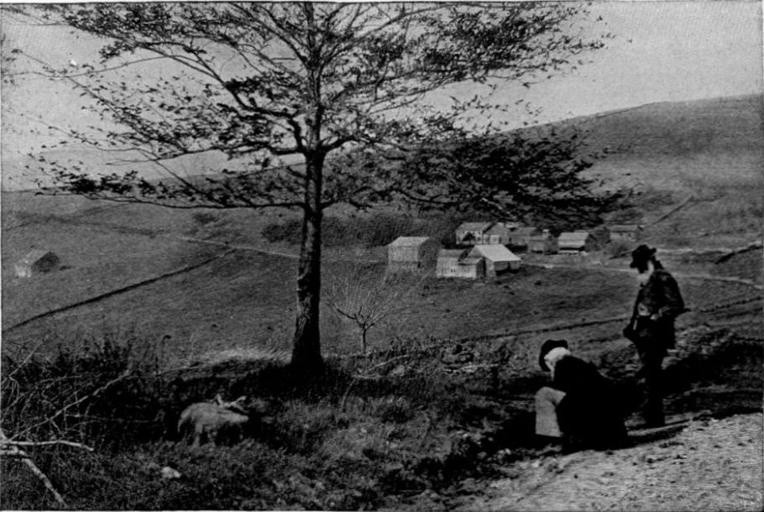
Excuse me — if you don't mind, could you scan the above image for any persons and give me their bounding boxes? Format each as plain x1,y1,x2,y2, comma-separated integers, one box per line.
622,245,683,429
535,339,630,456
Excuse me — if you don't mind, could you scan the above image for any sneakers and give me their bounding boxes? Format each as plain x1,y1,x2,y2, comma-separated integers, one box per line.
536,443,562,457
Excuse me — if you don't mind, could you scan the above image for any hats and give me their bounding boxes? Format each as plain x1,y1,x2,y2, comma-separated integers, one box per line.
539,339,568,372
629,246,657,269
